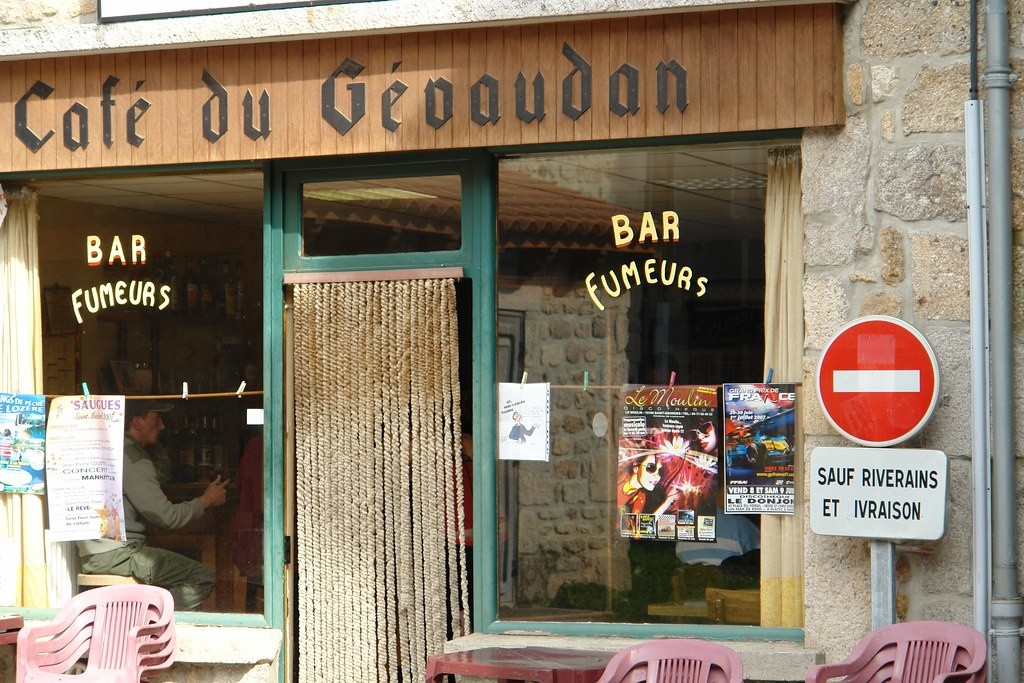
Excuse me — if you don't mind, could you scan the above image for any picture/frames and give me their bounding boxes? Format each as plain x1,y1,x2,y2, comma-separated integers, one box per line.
110,359,141,395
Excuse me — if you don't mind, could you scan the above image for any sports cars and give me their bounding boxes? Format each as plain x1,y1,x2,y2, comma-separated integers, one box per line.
727,436,791,467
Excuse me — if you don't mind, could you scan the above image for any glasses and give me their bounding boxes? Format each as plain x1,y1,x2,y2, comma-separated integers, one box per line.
638,463,664,477
683,420,713,442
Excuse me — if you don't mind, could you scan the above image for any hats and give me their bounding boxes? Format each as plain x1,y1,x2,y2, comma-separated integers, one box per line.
126,399,175,421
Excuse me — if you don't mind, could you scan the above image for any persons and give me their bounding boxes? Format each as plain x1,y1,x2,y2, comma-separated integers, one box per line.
409,433,473,547
617,417,717,523
675,468,760,566
76,390,231,611
232,436,265,571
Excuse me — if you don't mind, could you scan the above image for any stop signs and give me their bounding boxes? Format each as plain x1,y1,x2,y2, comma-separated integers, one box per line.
814,312,941,450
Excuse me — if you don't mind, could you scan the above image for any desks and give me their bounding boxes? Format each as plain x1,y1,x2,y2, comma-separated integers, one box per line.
425,646,618,683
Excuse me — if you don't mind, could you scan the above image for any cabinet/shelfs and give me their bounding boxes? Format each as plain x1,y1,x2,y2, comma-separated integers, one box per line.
95,307,243,482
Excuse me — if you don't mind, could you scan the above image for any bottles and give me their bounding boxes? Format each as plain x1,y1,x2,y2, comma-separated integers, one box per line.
146,249,246,319
243,341,256,387
166,414,224,481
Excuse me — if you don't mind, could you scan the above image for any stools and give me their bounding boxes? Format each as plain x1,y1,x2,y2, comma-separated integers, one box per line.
76,573,143,593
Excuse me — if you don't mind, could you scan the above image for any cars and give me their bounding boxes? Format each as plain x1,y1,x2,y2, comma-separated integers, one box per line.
641,520,653,529
678,514,694,524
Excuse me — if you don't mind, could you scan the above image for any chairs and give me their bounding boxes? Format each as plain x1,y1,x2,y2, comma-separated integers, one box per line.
15,584,178,683
596,639,743,683
805,620,987,683
